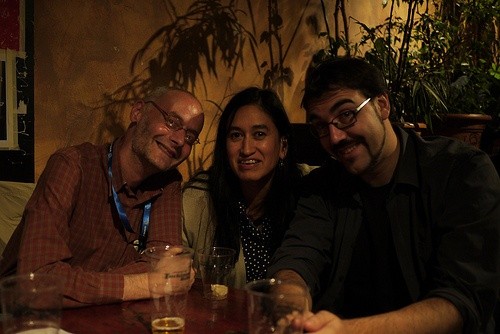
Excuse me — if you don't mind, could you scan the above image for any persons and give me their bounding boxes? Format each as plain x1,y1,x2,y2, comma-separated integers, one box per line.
266,55,500,334
180,87,304,291
0,86,204,309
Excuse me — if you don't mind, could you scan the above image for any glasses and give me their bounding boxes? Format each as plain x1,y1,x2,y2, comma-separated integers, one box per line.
144,100,202,145
311,98,372,138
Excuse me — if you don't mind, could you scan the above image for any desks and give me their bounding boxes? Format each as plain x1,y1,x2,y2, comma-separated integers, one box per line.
1,280,273,334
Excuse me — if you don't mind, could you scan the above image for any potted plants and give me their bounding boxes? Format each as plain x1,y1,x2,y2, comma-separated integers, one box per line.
170,0,500,166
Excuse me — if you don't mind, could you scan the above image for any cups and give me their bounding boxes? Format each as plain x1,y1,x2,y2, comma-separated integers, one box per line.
0,273,62,334
196,246,236,300
145,246,194,334
246,278,310,334
